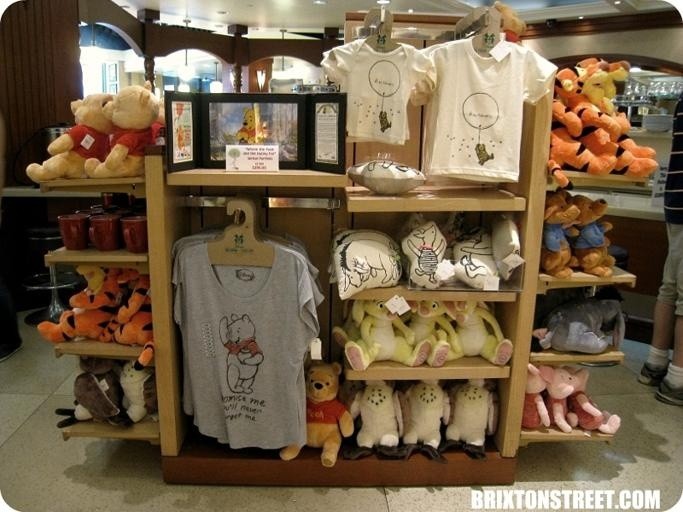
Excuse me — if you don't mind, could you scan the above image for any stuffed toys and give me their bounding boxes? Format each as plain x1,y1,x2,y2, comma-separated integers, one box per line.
35,266,160,426
519,55,659,434
326,213,520,449
279,362,355,467
25,78,160,426
520,54,658,433
326,213,521,450
25,80,159,186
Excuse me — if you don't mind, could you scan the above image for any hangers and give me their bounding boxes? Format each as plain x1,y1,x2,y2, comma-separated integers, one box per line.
206,192,291,267
452,6,502,56
360,7,400,51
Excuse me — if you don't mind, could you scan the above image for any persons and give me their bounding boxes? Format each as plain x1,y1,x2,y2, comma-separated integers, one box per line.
637,90,683,406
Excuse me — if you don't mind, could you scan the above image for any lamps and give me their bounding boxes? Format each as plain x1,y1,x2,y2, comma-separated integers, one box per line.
80,5,288,94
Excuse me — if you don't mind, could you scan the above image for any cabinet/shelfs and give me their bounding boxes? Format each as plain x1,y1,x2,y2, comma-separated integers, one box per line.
40,1,650,487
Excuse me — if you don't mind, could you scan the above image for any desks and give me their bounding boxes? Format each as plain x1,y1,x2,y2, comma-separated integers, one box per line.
0,185,102,198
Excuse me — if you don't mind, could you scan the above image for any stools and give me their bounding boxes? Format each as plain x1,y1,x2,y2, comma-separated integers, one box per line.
24,227,87,329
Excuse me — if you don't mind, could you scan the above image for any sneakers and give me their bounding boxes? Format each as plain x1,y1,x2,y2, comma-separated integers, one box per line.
638,358,672,387
654,376,682,407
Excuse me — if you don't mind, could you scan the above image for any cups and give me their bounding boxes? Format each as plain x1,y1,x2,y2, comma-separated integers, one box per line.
88,214,123,251
58,214,91,251
120,216,149,253
76,192,147,215
